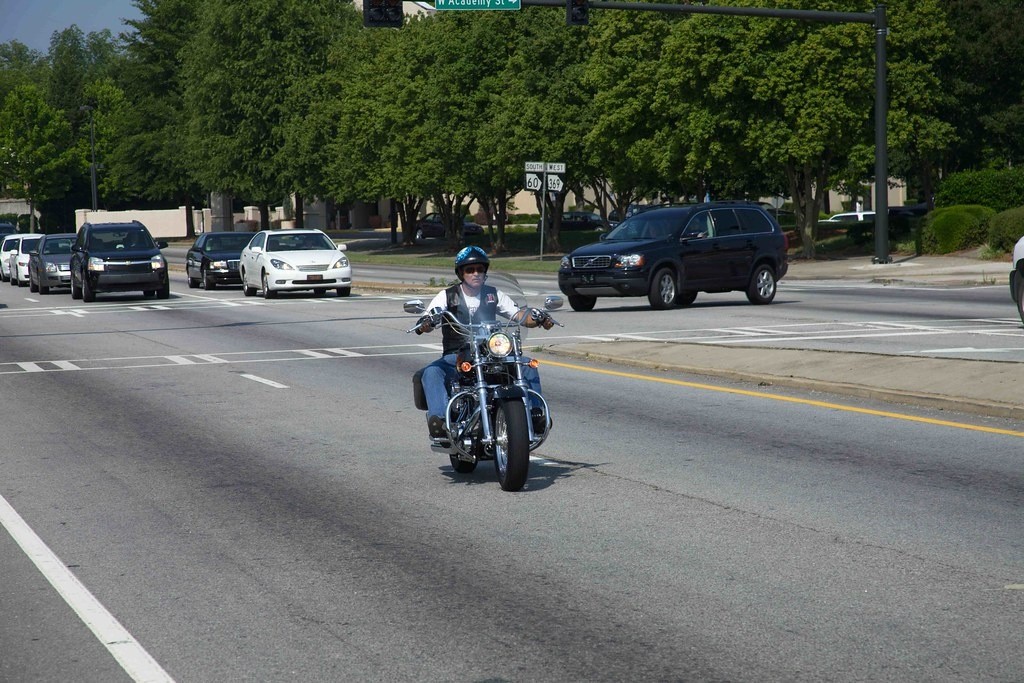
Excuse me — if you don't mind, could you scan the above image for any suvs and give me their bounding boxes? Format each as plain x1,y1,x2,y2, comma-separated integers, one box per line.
557,202,789,314
67,219,170,303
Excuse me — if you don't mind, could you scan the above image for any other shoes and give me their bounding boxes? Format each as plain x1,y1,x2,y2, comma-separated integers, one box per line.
530,407,553,435
428,415,451,448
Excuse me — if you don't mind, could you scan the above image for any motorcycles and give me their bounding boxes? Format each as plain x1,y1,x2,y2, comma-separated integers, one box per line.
403,270,565,493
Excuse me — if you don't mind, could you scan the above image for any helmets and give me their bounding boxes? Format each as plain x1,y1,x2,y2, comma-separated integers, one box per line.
455,245,490,281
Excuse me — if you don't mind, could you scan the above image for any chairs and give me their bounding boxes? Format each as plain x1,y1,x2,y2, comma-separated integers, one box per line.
270,241,279,251
92,238,105,249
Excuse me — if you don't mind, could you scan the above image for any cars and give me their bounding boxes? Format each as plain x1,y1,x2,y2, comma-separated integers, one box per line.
1009,236,1024,328
185,230,277,291
238,226,355,300
536,211,622,233
609,199,931,238
0,223,83,295
413,212,485,240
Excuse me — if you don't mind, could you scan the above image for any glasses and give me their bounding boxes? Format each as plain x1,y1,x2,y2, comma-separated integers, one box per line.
463,266,486,274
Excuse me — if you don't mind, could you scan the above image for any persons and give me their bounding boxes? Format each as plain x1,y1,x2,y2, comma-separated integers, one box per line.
648,224,660,238
856,201,862,212
212,238,224,250
415,246,554,448
689,218,704,232
301,237,319,248
125,232,146,248
